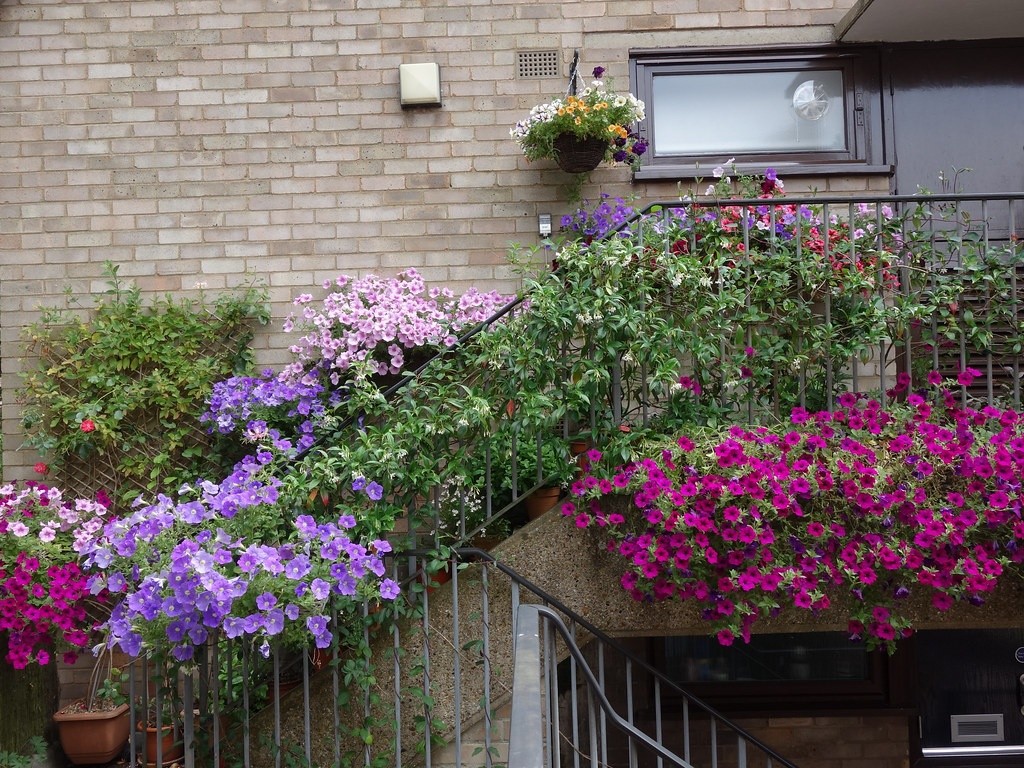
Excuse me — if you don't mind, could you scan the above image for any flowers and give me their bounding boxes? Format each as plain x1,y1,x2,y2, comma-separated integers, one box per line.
509,66,650,206
0,152,1024,768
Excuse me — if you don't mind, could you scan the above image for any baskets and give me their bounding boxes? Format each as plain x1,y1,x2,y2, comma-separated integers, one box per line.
550,128,609,174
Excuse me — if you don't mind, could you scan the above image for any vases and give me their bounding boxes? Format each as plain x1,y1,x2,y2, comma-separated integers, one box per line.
571,441,589,478
266,674,300,707
416,562,451,593
527,487,560,521
137,720,184,763
53,695,130,765
193,703,229,749
470,535,501,562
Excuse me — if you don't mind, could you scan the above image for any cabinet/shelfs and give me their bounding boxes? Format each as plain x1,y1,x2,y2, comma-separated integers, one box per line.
634,632,921,722
